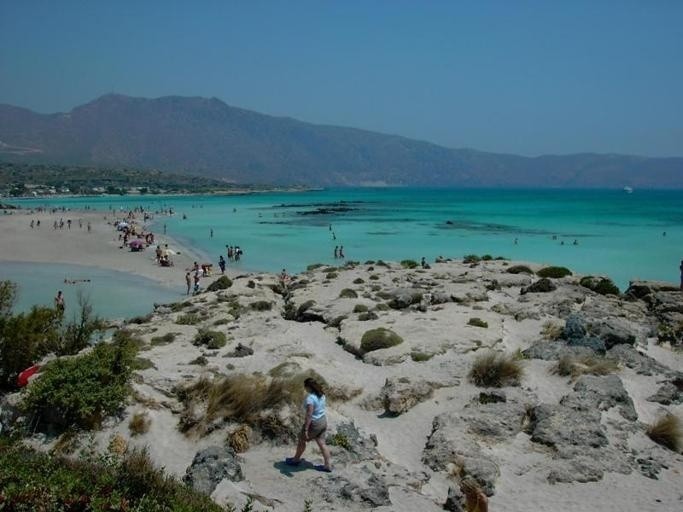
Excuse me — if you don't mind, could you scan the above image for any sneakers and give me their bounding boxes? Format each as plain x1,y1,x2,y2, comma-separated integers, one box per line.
286,457,301,466
314,463,333,472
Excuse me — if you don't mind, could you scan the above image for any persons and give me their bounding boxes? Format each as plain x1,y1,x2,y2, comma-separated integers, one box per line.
54,290,64,324
338,246,344,258
225,244,231,258
280,269,288,279
230,246,235,262
191,262,199,276
113,208,171,267
192,272,200,290
219,255,225,275
334,246,339,257
185,272,192,295
286,377,332,472
235,246,240,263
201,263,212,277
29,206,112,230
